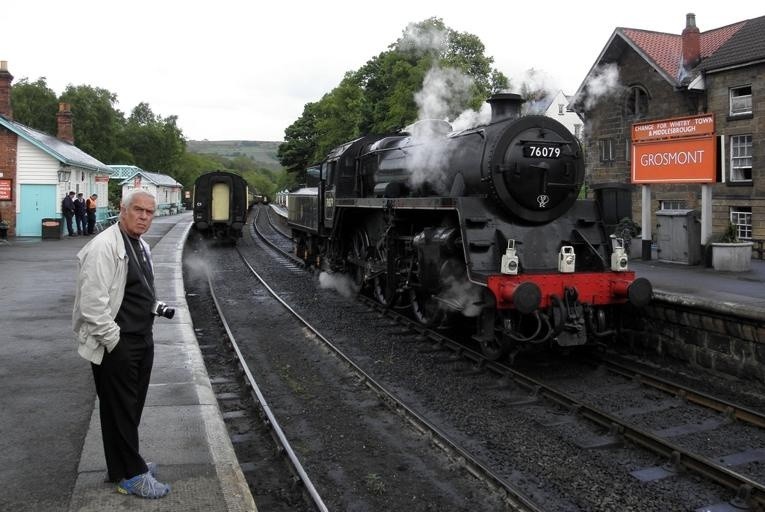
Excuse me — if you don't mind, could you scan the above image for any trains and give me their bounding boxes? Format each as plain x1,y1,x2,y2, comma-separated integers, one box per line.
281,92,654,367
189,169,269,245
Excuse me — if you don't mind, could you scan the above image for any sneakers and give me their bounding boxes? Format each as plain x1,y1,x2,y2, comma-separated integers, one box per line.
117,462,171,499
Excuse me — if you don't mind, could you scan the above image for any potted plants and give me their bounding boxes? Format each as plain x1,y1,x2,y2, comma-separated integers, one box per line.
704,222,754,273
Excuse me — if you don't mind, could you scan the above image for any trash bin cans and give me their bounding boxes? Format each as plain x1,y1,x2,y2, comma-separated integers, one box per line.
40,218,64,240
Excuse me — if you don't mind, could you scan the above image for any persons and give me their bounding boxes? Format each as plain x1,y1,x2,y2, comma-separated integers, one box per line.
74,193,90,236
87,193,98,234
72,188,181,500
61,192,75,237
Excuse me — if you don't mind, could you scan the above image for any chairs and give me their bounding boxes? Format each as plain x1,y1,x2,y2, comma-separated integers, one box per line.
93,209,119,233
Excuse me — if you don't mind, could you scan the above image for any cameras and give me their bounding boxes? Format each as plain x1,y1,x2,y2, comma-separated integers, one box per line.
151,302,175,319
